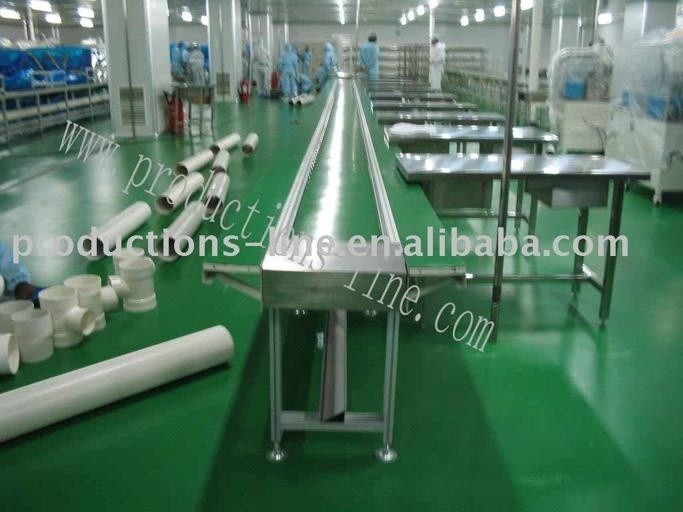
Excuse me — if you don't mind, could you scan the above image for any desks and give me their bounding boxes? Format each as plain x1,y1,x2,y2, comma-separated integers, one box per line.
361,78,651,329
0,83,110,156
173,84,215,134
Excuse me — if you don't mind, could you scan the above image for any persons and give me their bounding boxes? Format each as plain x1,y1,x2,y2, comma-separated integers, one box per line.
253,38,272,98
295,45,313,77
425,35,447,93
360,32,380,100
186,40,205,84
275,43,300,99
315,61,329,86
321,41,337,73
295,73,314,94
179,45,192,74
169,41,187,85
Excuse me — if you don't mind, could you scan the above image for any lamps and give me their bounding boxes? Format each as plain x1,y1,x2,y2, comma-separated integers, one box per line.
400,0,534,27
0,0,209,30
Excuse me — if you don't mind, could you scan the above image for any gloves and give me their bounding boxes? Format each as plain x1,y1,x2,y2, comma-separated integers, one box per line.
15,281,46,308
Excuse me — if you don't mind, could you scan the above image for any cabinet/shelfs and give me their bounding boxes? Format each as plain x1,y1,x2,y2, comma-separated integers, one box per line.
346,45,486,77
